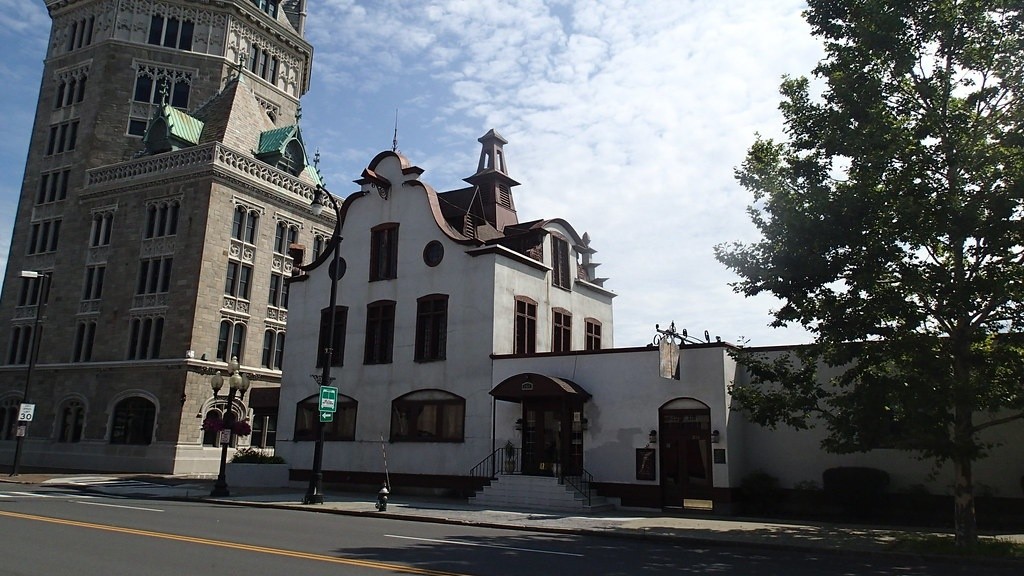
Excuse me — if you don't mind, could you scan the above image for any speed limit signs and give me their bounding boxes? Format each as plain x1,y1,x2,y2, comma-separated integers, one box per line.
18,403,36,422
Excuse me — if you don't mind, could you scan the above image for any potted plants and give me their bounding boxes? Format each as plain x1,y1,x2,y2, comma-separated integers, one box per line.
504,440,516,474
225,448,290,496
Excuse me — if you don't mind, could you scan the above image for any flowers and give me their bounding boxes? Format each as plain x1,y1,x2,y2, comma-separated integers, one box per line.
199,417,251,437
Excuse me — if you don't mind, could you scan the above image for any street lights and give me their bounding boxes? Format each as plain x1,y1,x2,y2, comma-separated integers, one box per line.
209,354,251,497
5,269,50,478
301,181,344,504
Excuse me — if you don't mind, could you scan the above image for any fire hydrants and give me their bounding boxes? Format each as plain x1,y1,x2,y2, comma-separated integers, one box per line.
375,486,390,511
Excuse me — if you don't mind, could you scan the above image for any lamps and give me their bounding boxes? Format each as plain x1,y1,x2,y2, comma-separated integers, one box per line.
648,430,657,443
515,418,522,430
581,418,588,430
710,430,719,443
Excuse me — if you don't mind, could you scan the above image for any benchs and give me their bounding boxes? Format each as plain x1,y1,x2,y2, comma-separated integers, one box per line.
683,498,713,516
